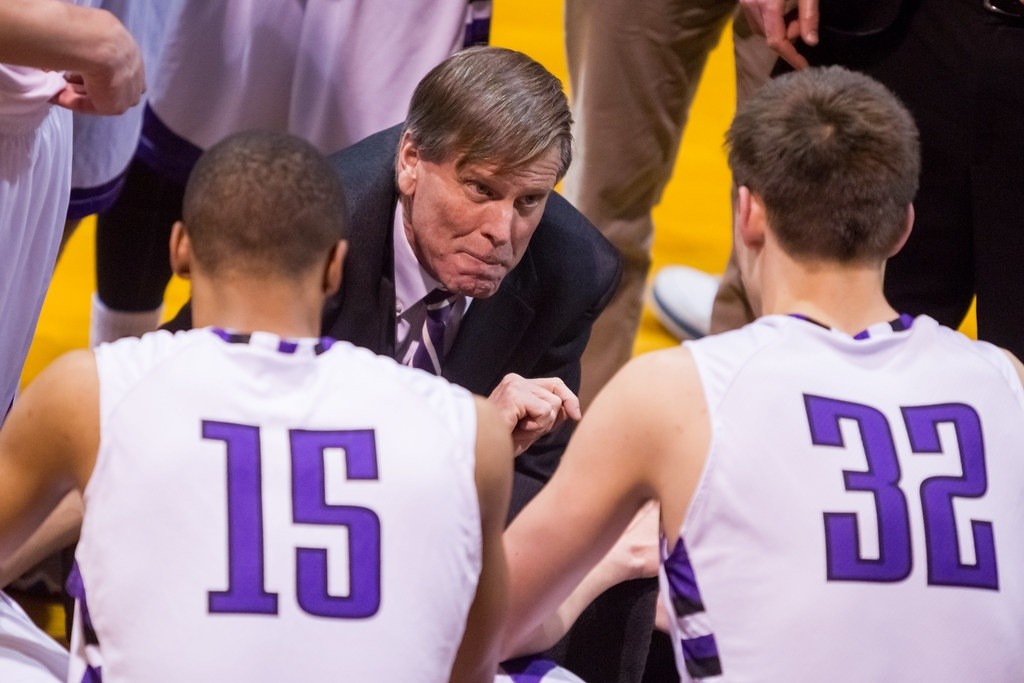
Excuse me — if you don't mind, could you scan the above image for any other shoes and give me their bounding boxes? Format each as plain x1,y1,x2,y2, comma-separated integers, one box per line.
651,262,723,342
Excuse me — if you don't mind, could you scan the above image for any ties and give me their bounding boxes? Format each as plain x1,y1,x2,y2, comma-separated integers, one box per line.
406,288,460,377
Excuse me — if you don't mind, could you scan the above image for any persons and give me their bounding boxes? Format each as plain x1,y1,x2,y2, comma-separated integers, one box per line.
323,45,660,683
1,0,1024,683
